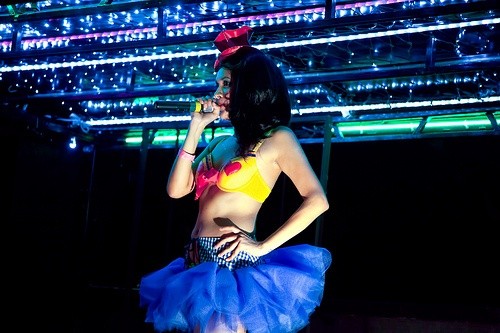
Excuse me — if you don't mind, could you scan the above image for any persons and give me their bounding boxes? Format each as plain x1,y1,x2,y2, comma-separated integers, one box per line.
168,45,331,333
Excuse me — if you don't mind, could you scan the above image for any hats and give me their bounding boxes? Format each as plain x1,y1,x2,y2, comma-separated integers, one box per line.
213,25,251,72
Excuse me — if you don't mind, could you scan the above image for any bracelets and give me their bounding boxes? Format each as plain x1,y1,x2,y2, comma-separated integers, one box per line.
179,148,196,156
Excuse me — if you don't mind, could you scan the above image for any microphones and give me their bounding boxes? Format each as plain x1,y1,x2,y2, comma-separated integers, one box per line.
154,101,215,113
